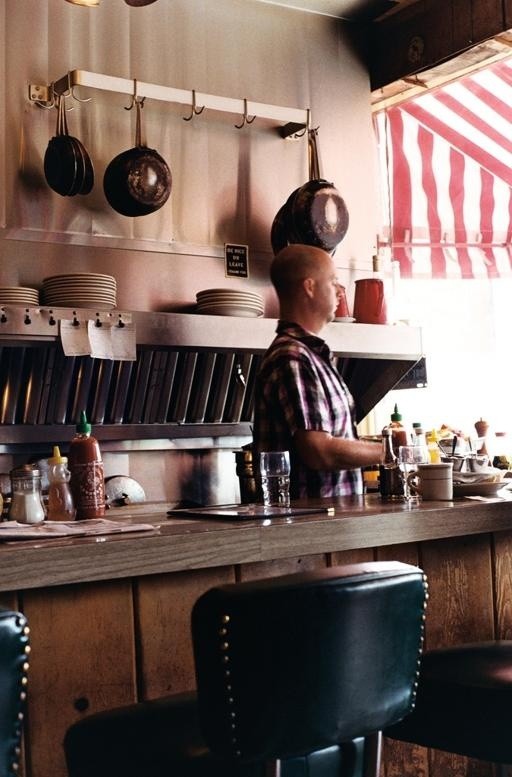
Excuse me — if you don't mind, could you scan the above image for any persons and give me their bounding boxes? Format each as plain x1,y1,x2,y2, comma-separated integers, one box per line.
249,242,412,501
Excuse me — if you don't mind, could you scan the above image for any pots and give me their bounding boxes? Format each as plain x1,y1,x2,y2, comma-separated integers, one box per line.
101,99,174,218
42,97,97,199
270,122,349,262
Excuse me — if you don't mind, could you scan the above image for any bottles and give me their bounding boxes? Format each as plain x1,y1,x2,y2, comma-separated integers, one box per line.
44,444,76,522
372,255,382,273
475,416,492,467
378,430,404,504
7,463,45,524
380,402,409,462
493,431,512,469
439,438,464,455
68,408,105,517
410,421,441,463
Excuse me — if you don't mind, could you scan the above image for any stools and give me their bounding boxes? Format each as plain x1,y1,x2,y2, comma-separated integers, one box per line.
379,636,512,765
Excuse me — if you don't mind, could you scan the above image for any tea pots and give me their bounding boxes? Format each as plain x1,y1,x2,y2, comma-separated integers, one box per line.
352,278,388,324
333,283,351,316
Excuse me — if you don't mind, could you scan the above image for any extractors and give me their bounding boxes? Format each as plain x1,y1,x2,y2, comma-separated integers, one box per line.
0,308,423,455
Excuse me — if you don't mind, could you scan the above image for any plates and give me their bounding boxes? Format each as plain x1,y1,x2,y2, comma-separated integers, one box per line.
330,315,359,323
0,286,40,306
194,288,265,318
454,480,510,495
452,472,499,483
42,272,118,310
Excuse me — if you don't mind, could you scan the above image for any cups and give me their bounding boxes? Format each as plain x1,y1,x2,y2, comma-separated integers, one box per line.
407,461,455,502
258,450,292,508
441,452,490,473
395,443,428,502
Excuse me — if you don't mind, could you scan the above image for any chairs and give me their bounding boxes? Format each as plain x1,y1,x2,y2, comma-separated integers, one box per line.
0,606,33,775
61,557,431,776
